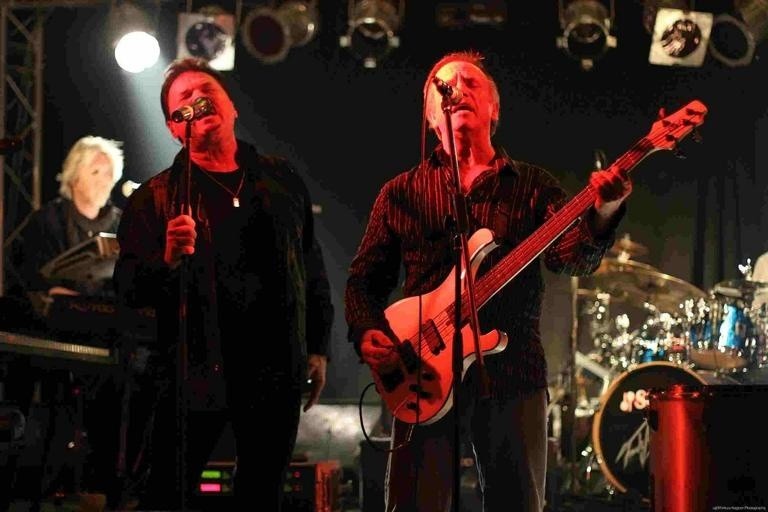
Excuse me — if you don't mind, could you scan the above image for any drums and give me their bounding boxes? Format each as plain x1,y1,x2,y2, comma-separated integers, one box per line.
592,360,741,504
679,294,757,373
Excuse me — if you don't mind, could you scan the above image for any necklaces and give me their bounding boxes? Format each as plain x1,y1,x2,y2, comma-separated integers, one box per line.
197,165,248,207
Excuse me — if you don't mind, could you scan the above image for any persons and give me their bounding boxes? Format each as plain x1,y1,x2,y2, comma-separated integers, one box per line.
111,57,335,511
342,49,635,512
2,132,127,494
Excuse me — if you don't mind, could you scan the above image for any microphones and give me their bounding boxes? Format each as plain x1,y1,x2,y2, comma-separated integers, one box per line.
120,179,142,198
431,74,464,106
171,94,214,123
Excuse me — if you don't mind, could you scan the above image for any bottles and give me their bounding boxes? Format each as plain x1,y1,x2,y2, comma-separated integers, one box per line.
460,458,482,511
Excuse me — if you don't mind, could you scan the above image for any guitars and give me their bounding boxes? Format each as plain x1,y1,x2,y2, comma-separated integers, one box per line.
369,99,708,427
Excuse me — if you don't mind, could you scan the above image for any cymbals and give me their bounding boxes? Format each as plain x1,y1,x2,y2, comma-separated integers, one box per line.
591,270,707,312
594,257,655,271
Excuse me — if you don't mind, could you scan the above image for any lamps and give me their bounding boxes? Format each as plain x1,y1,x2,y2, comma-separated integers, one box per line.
174,1,237,74
640,1,715,71
556,0,618,70
708,2,767,69
340,1,408,72
241,0,318,67
98,0,161,73
